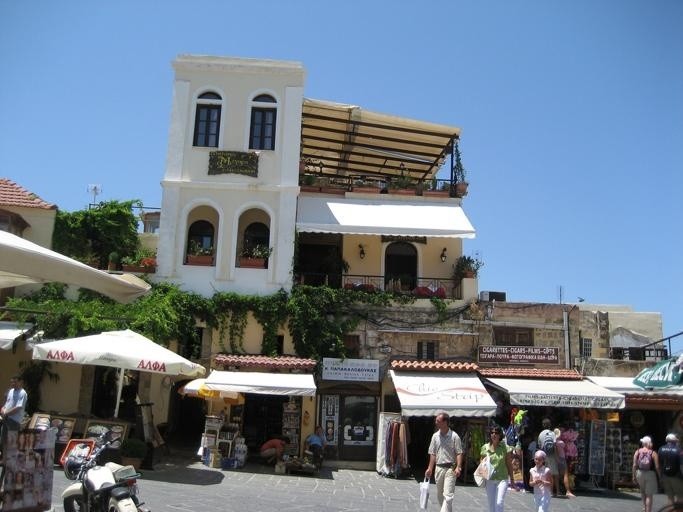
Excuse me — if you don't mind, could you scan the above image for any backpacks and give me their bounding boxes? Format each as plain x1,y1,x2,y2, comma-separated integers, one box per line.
542,436,555,456
637,450,652,471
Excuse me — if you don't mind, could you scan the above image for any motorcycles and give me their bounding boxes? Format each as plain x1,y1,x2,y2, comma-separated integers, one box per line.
59,431,150,511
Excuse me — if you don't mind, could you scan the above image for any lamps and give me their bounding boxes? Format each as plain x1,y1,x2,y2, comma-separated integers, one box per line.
438,247,449,264
356,243,366,259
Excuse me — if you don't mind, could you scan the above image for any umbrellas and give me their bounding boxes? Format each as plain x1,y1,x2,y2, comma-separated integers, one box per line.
0,227,152,308
177,376,245,416
30,329,207,421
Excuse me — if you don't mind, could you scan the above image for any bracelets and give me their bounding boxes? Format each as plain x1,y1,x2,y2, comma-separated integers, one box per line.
509,477,514,480
456,464,462,470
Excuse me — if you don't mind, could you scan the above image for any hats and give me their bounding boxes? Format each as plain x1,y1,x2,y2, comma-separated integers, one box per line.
665,433,680,442
639,436,651,444
534,450,547,459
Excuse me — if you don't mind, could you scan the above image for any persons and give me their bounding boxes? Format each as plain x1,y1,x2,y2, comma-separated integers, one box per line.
0,375,30,466
629,435,660,512
259,435,289,466
527,431,539,490
3,431,52,509
552,427,576,500
528,450,553,512
656,433,682,505
304,425,328,470
424,411,463,512
536,418,565,498
477,425,514,512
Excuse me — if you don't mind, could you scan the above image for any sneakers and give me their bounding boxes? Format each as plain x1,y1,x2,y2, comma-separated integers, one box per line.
566,491,577,498
550,493,564,498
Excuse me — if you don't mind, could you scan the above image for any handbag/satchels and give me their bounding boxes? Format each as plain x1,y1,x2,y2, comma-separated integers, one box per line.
472,457,495,487
419,476,431,511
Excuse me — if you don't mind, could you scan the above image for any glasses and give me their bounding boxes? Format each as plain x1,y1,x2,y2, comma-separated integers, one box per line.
490,430,500,435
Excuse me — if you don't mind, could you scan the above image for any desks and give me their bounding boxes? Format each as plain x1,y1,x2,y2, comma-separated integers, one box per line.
341,280,376,294
409,286,448,300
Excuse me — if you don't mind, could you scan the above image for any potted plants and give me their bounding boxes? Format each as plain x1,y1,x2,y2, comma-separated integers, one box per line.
119,438,148,473
182,237,215,267
298,152,469,199
103,246,156,276
235,238,275,269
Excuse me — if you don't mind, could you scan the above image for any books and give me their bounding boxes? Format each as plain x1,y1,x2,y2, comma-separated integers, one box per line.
283,414,299,457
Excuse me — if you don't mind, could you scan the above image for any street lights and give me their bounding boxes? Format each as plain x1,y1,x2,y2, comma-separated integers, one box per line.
566,295,584,368
